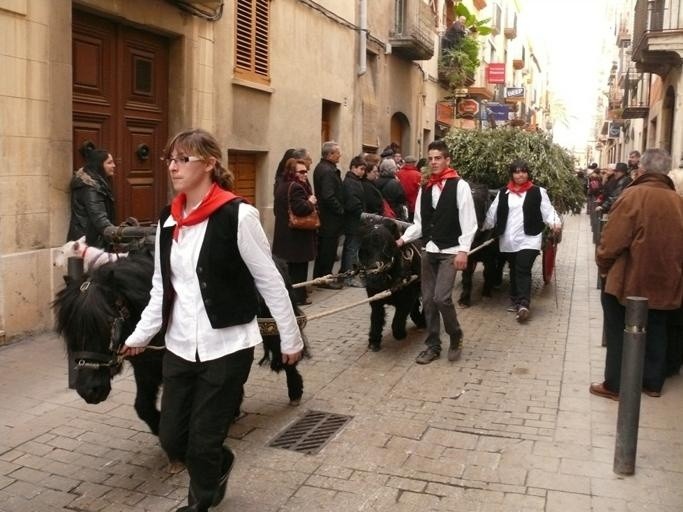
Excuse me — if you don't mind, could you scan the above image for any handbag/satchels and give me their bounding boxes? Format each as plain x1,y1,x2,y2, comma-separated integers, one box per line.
382,199,394,215
287,204,321,230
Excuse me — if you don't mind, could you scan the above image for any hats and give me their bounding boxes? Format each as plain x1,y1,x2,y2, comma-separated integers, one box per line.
364,149,417,166
611,163,628,172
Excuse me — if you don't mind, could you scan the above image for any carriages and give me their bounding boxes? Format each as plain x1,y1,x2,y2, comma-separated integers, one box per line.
49,169,566,468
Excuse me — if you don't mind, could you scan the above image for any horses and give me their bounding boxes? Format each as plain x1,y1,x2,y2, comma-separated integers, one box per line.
47,238,307,476
356,216,431,353
455,184,510,309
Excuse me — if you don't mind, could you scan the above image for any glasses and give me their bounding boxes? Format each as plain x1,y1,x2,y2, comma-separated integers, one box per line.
295,158,311,174
165,155,208,166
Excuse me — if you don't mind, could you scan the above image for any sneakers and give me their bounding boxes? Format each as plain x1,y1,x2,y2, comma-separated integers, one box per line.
209,444,235,507
414,346,440,364
446,332,462,361
506,300,529,323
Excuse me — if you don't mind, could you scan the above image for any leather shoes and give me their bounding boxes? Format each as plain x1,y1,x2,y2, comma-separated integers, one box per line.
640,388,660,397
293,285,312,305
312,277,365,290
588,382,619,401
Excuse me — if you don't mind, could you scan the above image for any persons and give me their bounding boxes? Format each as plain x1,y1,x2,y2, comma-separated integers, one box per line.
481,159,561,324
589,148,683,213
395,141,477,364
65,137,118,252
120,126,306,512
589,147,683,398
271,140,427,306
